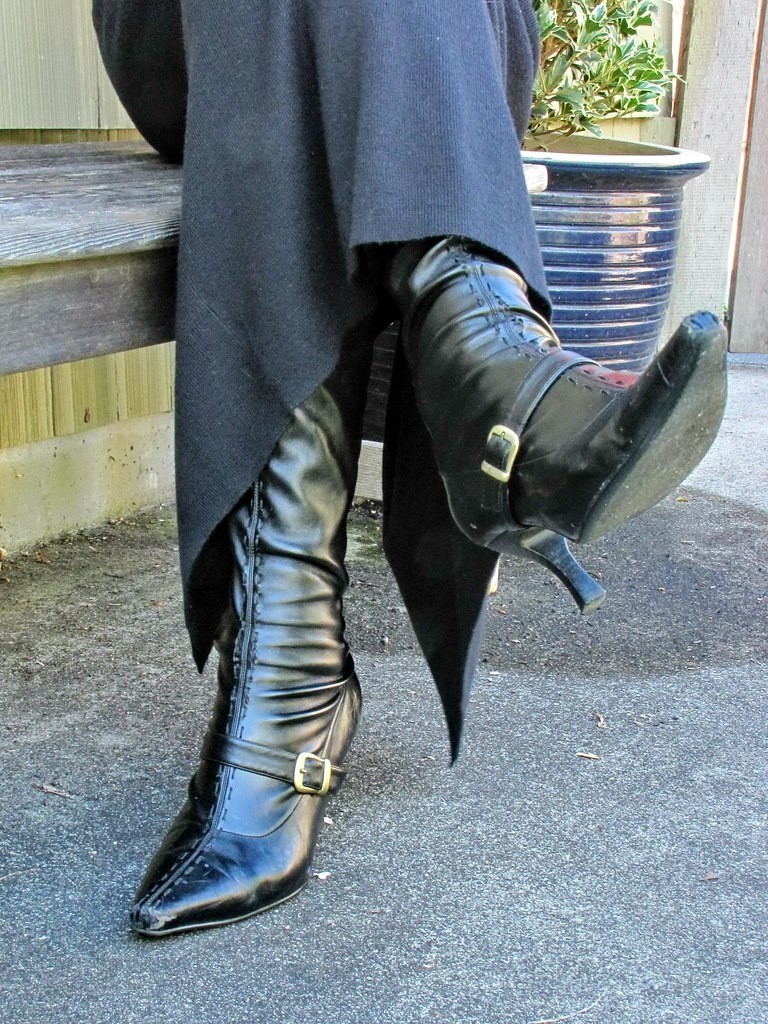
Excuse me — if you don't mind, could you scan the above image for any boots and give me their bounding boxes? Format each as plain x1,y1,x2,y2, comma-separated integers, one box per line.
392,234,727,612
129,378,365,936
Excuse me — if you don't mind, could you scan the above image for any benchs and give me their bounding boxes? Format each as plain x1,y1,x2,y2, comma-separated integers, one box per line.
0,0,547,376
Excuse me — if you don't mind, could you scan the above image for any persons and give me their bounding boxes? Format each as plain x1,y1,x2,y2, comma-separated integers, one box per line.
90,0,729,937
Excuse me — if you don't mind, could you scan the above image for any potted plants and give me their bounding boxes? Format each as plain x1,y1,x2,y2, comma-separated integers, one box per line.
521,1,709,375
